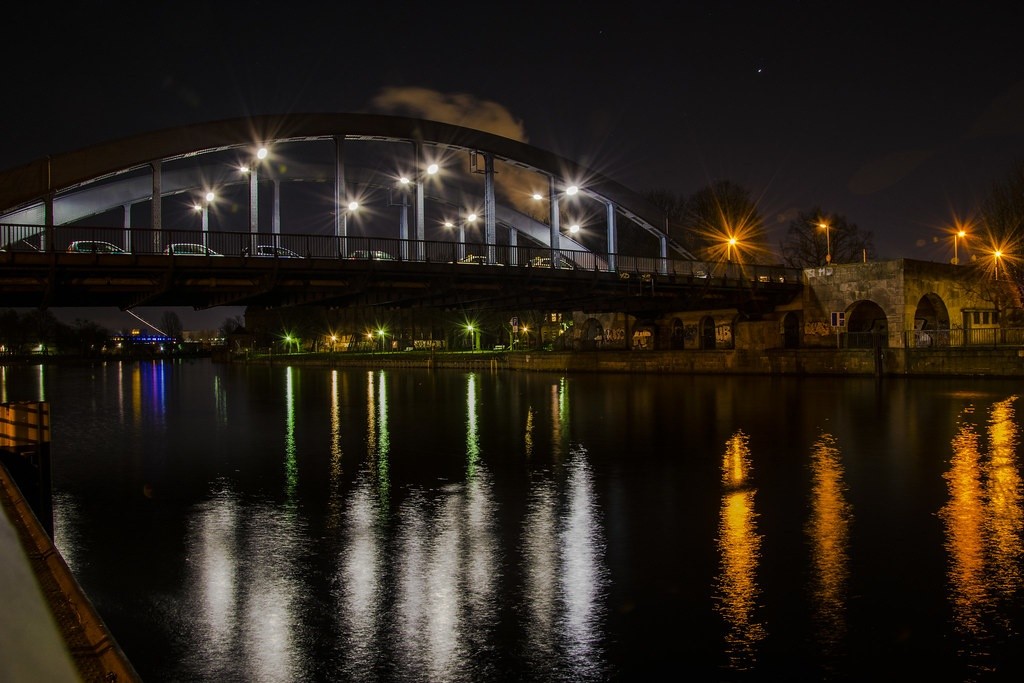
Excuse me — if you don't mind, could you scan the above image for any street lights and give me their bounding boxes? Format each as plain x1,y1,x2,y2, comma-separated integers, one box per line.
820,224,830,266
468,326,474,351
955,231,966,265
728,239,735,260
379,330,385,351
994,251,1001,280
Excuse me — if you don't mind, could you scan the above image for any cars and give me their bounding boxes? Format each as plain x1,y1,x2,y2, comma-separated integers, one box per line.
163,243,224,256
448,256,504,266
525,257,573,270
244,246,306,258
348,250,398,261
68,240,132,255
